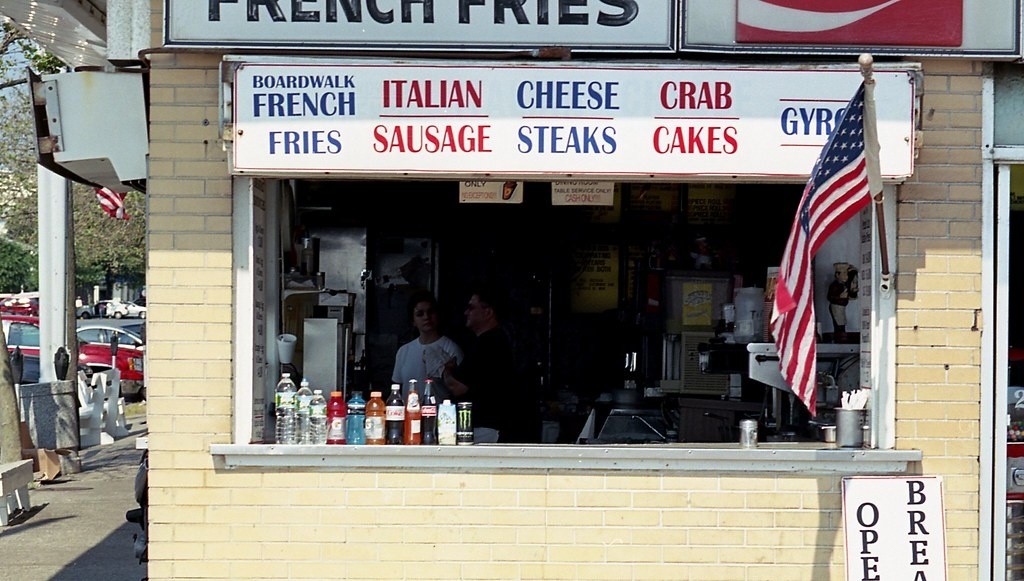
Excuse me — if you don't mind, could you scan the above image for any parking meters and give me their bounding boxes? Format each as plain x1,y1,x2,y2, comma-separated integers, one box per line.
110,330,118,369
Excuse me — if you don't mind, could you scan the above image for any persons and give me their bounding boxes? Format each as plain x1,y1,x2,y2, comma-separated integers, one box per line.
442,291,544,442
386,290,466,402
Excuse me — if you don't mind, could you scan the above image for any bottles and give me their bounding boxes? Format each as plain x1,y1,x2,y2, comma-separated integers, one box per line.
405,379,422,445
384,384,406,445
296,382,313,444
347,391,367,445
327,392,347,444
274,372,297,445
420,380,438,445
365,392,386,445
309,390,327,445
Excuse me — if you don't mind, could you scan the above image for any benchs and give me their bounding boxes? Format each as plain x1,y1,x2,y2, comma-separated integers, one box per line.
78,368,121,445
76,370,127,429
0,458,34,524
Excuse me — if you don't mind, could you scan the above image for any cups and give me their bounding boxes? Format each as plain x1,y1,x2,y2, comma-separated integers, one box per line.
730,373,742,397
275,333,298,364
600,394,610,400
833,407,867,447
763,272,781,342
646,387,662,397
734,320,754,344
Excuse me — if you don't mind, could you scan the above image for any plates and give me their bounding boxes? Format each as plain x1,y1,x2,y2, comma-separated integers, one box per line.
600,399,612,402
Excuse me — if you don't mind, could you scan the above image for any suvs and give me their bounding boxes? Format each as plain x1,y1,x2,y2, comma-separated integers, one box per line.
0,314,145,401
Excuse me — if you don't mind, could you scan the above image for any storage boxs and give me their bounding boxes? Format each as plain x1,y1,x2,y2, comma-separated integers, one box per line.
664,275,735,335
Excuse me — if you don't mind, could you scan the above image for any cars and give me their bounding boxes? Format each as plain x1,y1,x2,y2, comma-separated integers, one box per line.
76,324,144,353
95,300,145,319
0,296,38,315
76,308,93,320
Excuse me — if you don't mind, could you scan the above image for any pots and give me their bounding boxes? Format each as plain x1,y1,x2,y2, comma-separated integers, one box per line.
703,412,761,425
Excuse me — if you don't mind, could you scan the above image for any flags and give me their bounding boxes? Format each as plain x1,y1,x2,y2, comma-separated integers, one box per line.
768,79,885,418
93,185,131,222
